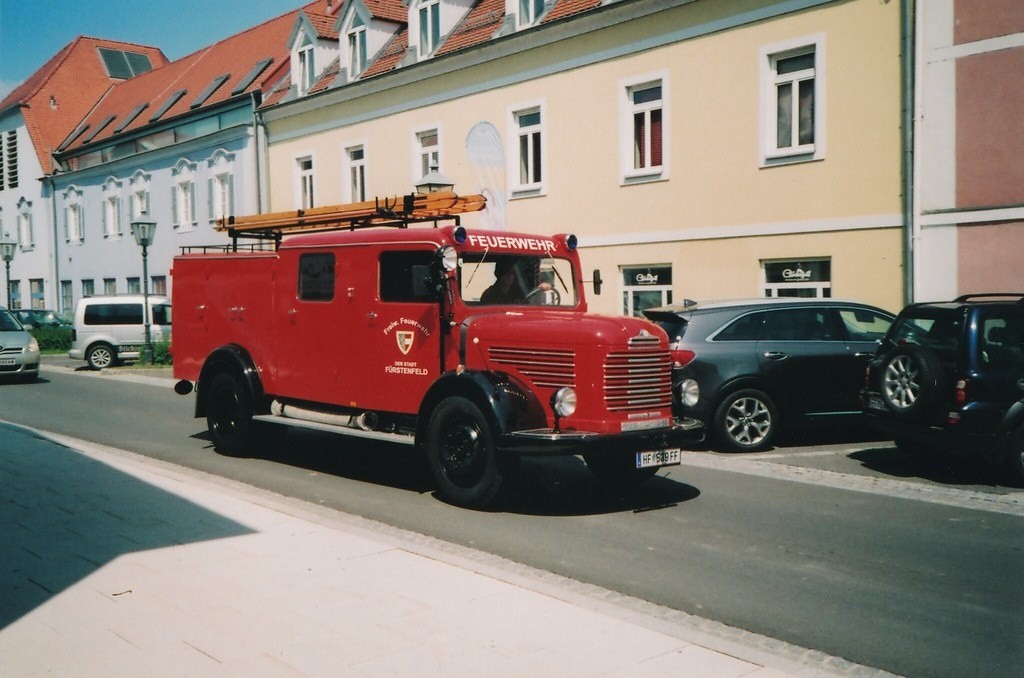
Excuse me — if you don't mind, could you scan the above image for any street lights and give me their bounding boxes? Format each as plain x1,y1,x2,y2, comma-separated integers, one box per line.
0,230,18,312
130,210,159,365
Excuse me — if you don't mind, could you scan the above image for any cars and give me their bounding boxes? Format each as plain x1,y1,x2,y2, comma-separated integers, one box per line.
0,304,41,382
10,308,75,344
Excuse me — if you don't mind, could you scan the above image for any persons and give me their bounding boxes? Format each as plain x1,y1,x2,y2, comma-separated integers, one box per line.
480,257,552,304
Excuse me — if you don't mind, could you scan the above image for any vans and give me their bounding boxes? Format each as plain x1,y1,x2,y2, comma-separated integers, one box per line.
67,292,172,371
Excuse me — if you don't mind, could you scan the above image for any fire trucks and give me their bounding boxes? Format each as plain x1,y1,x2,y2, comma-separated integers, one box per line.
163,189,708,510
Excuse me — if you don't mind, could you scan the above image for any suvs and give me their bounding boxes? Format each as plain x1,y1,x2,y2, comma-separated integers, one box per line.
858,291,1024,484
640,297,932,453
